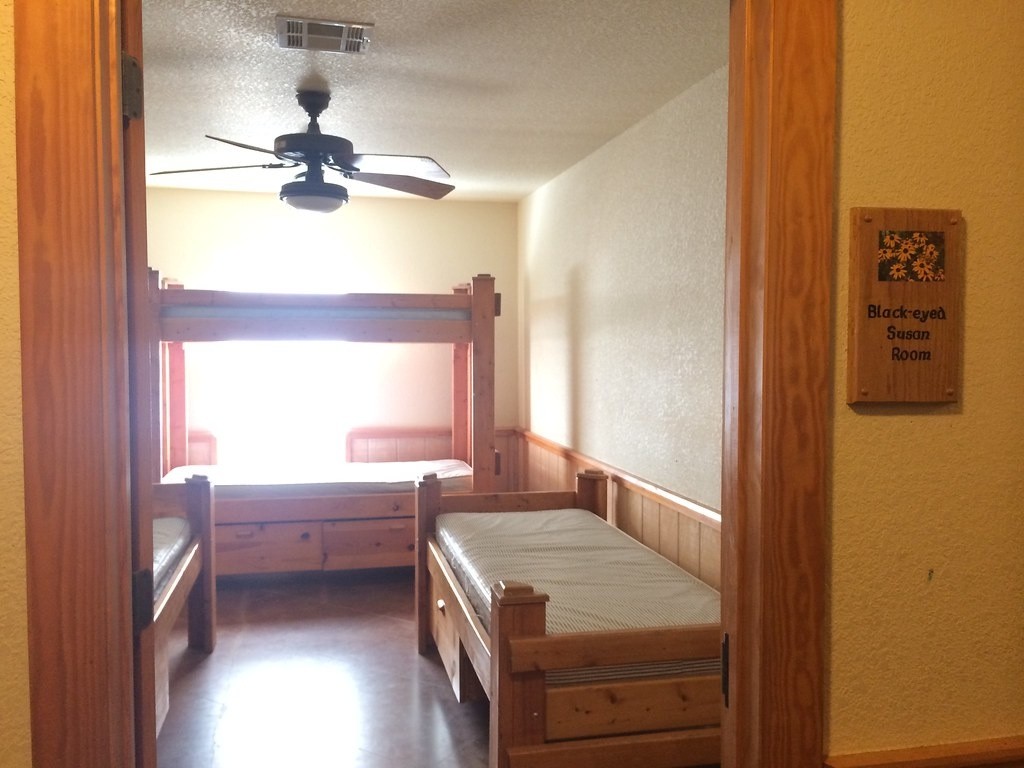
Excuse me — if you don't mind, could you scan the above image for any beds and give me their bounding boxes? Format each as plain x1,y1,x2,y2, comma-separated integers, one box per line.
146,268,502,575
416,467,725,768
150,475,214,732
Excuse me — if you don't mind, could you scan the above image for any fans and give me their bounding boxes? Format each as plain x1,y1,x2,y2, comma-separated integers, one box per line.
151,90,454,201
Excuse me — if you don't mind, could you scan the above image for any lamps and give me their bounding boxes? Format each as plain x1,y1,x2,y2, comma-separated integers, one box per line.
281,166,350,212
